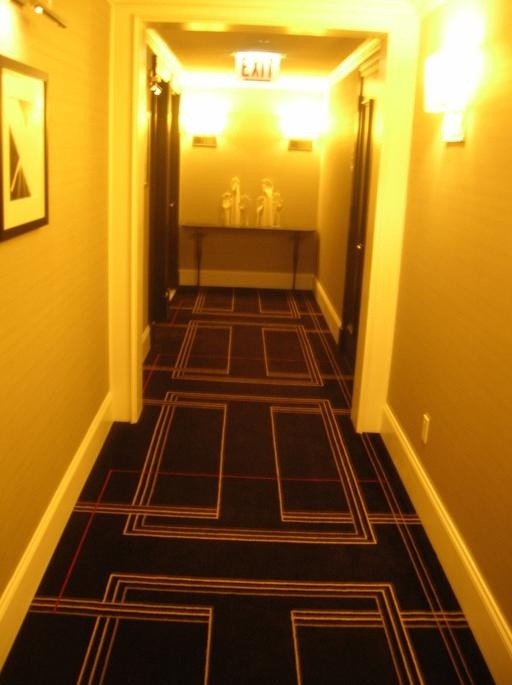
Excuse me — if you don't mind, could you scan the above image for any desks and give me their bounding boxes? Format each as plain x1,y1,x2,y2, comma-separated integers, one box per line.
181,222,317,291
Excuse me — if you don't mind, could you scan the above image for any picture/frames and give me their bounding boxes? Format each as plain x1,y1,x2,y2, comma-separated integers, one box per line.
0,55,49,242
